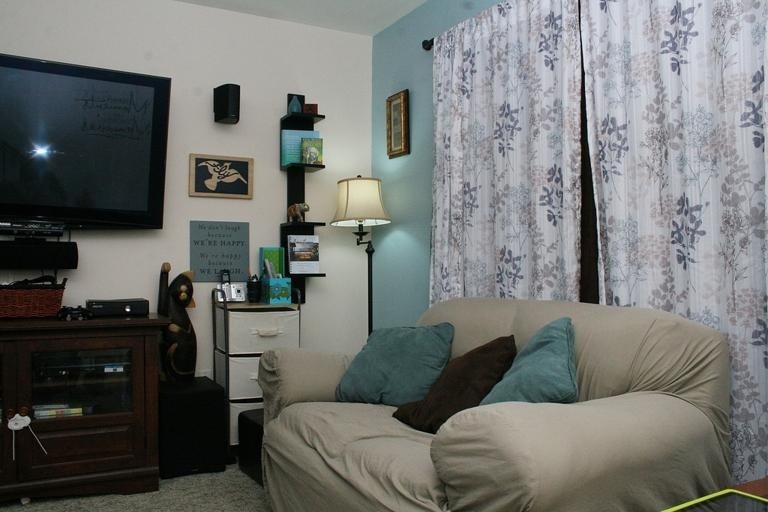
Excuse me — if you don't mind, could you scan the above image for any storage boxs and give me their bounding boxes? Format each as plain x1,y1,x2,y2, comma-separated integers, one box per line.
212,288,302,464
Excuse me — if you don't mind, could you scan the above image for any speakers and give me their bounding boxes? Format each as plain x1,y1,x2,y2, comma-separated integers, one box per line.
158,378,227,478
0,240,79,270
214,84,240,124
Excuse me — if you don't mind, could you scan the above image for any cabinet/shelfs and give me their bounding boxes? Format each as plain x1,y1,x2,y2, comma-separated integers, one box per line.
280,94,324,304
0,314,171,503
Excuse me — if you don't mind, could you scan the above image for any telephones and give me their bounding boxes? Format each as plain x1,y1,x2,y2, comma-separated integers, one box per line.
217,268,245,303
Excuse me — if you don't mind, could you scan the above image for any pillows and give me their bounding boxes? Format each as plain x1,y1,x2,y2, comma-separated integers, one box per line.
392,335,517,434
335,322,455,407
479,317,579,405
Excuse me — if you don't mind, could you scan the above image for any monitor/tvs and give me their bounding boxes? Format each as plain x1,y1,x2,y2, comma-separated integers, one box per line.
0,53,171,229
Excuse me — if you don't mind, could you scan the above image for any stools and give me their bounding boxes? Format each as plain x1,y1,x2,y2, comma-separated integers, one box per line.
159,377,225,480
238,409,264,487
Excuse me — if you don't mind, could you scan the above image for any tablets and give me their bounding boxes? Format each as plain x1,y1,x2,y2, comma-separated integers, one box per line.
661,489,768,512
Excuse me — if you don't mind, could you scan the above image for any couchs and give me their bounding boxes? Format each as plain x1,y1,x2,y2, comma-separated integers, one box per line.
258,298,731,512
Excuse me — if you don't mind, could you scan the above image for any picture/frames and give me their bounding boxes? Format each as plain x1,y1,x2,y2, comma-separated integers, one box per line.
189,154,254,200
386,89,409,159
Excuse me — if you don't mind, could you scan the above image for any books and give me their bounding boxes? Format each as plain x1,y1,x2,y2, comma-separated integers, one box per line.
280,129,320,167
300,138,323,165
287,234,320,274
32,403,82,420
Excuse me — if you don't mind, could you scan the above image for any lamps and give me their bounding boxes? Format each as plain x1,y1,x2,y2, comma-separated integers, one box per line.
330,175,391,335
